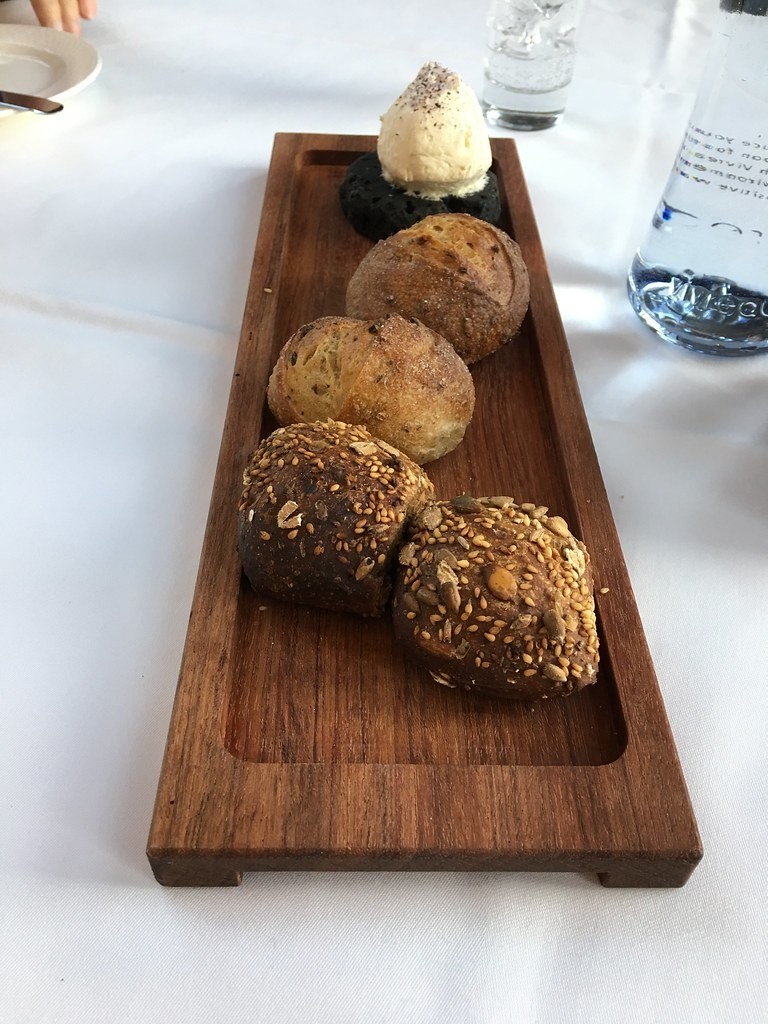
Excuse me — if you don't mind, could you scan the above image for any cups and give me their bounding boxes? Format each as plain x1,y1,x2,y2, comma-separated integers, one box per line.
479,0,587,132
628,0,768,358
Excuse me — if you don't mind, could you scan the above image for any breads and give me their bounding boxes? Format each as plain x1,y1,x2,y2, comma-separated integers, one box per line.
237,417,437,618
343,212,530,365
392,494,599,699
266,314,475,466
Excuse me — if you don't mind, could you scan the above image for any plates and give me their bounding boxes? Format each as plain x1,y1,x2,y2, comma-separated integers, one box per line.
0,23,100,122
145,134,706,888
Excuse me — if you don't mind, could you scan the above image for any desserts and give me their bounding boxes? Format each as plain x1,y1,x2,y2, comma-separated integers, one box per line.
340,59,501,239
